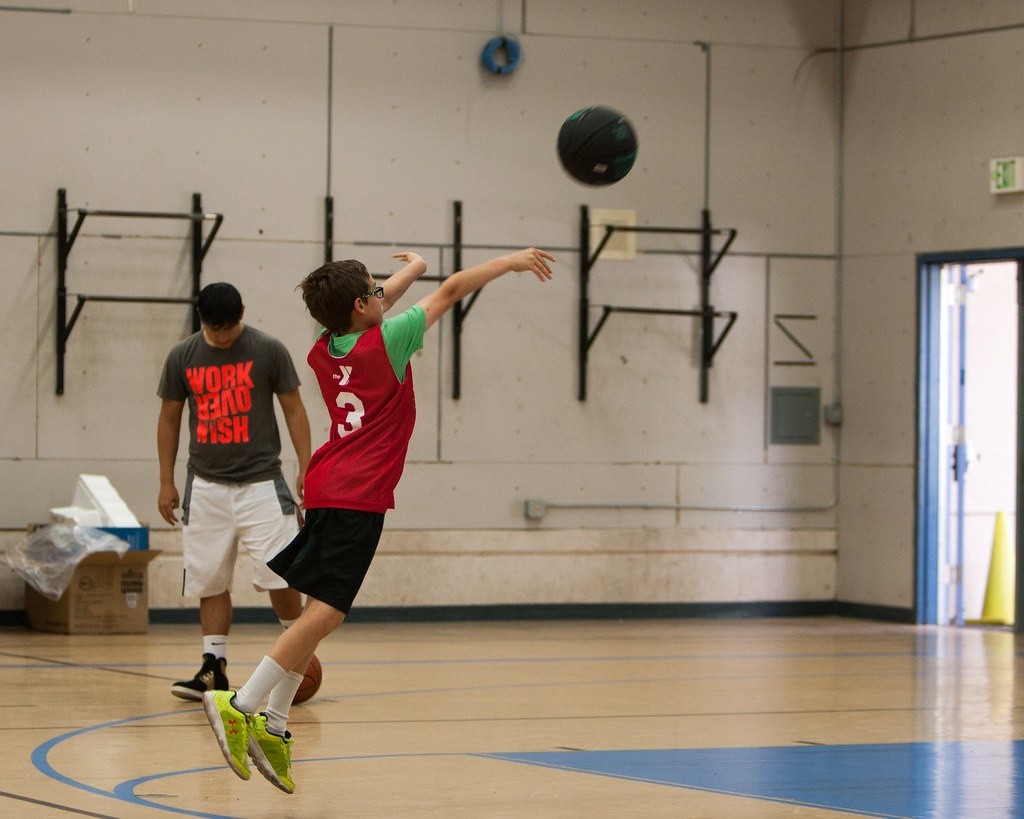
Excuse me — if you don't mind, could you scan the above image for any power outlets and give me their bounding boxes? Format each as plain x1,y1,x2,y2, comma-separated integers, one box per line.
526,499,548,520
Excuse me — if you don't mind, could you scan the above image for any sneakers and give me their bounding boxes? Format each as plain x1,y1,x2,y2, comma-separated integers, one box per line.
248,711,296,794
202,690,256,780
171,653,229,701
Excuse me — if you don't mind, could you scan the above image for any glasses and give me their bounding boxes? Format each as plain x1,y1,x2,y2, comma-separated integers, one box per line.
362,286,384,301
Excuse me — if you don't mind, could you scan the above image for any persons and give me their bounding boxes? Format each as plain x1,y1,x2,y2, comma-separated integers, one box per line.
156,282,312,702
204,248,556,794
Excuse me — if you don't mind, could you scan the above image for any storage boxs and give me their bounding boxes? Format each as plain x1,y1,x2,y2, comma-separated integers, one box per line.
24,522,164,633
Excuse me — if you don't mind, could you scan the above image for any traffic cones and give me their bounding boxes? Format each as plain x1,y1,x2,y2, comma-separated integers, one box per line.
976,512,1017,626
